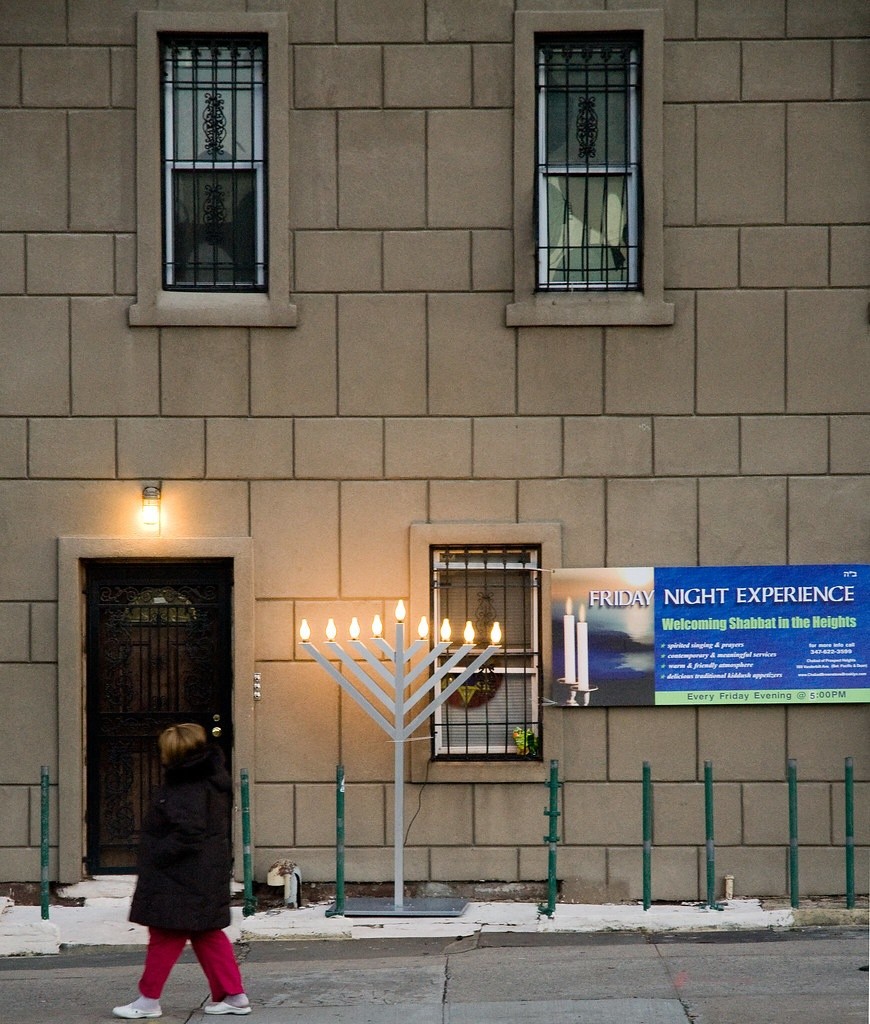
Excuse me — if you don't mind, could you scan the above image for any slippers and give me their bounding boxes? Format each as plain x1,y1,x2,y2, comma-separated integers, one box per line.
113,1003,162,1019
205,1002,252,1015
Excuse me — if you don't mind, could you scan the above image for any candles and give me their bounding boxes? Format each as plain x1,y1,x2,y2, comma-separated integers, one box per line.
576,604,589,691
563,597,575,683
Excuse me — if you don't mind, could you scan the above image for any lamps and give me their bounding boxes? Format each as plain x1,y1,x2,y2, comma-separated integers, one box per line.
141,493,161,526
300,599,502,916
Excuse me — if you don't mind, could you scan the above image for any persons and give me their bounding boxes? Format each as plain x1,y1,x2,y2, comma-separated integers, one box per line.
111,723,252,1019
